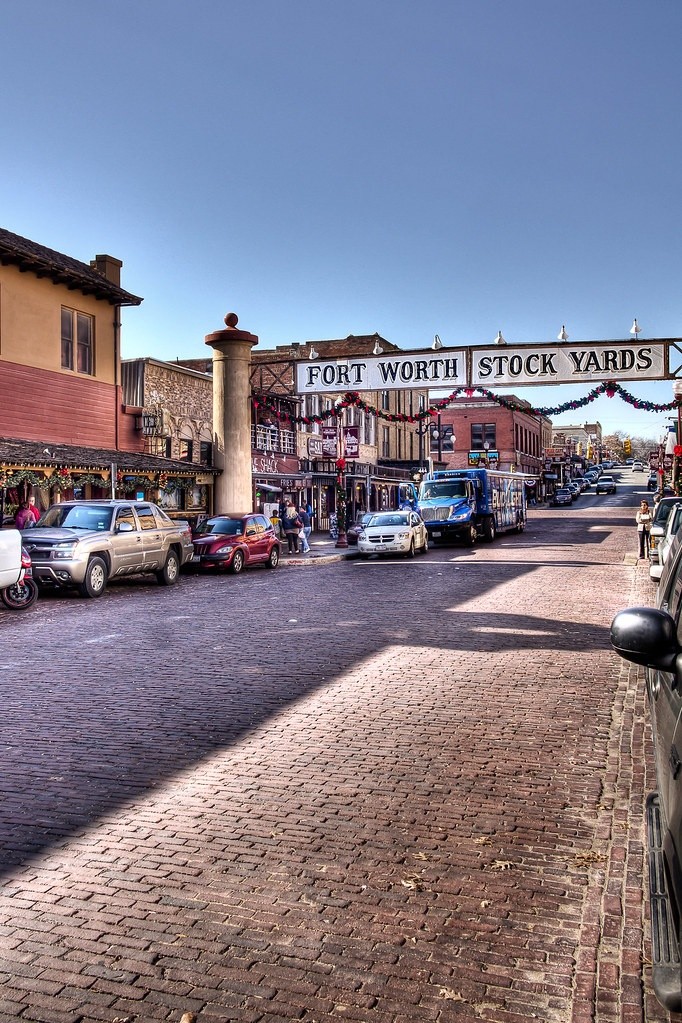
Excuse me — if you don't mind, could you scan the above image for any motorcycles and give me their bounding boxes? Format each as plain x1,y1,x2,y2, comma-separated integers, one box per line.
2,544,39,612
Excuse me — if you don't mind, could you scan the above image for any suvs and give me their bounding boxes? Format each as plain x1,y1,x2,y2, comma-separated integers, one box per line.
191,512,281,573
21,499,195,599
647,474,657,490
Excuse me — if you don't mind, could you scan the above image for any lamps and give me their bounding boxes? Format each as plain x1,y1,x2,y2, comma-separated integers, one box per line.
432,334,443,350
493,331,505,346
557,325,569,342
309,345,318,360
373,340,384,356
631,319,641,340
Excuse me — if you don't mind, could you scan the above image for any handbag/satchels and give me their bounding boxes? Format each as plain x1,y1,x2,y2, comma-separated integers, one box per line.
298,530,305,539
293,517,304,528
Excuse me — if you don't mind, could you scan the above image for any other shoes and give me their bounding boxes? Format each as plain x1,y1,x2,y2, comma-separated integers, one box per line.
639,555,645,559
303,547,310,554
295,549,300,554
288,551,293,554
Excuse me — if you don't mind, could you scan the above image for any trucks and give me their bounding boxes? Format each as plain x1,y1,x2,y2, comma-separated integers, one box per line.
397,468,528,544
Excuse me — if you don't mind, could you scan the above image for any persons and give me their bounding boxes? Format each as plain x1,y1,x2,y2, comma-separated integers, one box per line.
14,494,41,529
269,498,312,554
636,494,662,559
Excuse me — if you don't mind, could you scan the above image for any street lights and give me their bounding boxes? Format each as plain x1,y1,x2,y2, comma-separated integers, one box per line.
484,440,490,458
416,407,456,462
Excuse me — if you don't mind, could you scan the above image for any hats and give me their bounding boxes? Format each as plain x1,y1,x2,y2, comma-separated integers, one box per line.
288,503,294,507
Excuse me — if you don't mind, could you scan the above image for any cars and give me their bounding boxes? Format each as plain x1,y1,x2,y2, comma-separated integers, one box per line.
626,458,635,465
554,461,618,507
346,513,376,546
356,511,428,558
609,497,682,1023
632,461,644,472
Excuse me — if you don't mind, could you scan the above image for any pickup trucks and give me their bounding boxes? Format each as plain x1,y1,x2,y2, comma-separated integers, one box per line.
0,527,22,589
